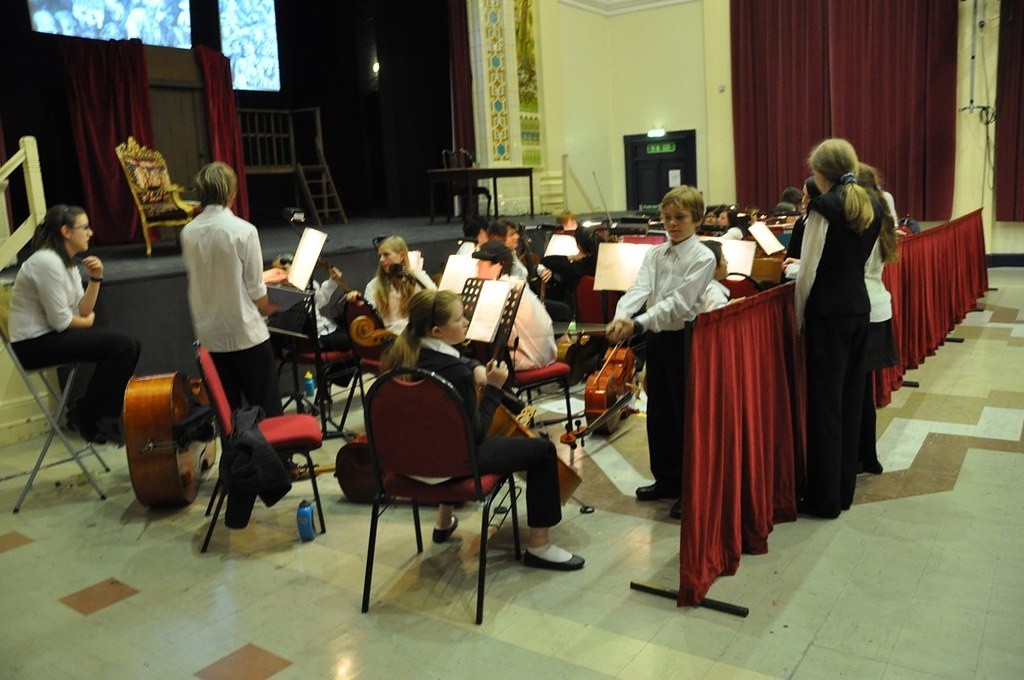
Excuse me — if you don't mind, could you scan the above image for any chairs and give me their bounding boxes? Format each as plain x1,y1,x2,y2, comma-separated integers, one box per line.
115,136,789,625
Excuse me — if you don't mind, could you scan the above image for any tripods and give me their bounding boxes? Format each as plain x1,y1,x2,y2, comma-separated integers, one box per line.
271,225,357,443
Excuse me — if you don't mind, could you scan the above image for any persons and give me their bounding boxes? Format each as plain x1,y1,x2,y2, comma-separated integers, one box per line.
773,138,900,521
366,234,440,335
271,253,351,350
605,185,756,527
382,287,587,570
179,161,299,469
7,205,143,445
438,211,603,374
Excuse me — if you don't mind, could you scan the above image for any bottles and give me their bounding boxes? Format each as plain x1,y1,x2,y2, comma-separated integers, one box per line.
297,499,317,541
304,370,314,397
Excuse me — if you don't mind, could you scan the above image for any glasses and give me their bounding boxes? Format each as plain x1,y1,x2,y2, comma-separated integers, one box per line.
70,224,90,232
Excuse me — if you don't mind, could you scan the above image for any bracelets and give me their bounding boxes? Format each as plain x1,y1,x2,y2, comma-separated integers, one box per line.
633,322,643,337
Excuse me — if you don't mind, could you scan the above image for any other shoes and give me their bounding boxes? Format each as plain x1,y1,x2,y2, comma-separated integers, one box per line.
432,515,458,544
856,461,883,475
66,408,106,444
670,499,682,519
797,496,851,519
635,482,678,501
523,547,586,570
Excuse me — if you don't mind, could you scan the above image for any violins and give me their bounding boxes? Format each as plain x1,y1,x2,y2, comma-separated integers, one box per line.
518,222,541,299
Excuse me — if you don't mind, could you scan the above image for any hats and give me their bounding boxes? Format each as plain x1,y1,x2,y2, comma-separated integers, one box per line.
472,241,513,264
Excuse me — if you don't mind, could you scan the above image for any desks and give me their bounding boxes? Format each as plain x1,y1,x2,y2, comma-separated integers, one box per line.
424,166,533,232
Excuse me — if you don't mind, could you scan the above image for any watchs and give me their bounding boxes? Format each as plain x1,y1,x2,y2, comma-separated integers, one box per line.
90,275,104,282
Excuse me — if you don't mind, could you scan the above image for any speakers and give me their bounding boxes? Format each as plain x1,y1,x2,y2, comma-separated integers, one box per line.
623,128,697,211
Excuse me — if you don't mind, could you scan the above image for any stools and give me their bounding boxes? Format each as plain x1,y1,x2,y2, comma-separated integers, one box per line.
0,306,110,513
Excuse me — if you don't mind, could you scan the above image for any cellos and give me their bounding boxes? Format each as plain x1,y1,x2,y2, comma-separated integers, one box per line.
349,315,587,509
560,347,642,449
123,369,218,510
555,334,600,387
